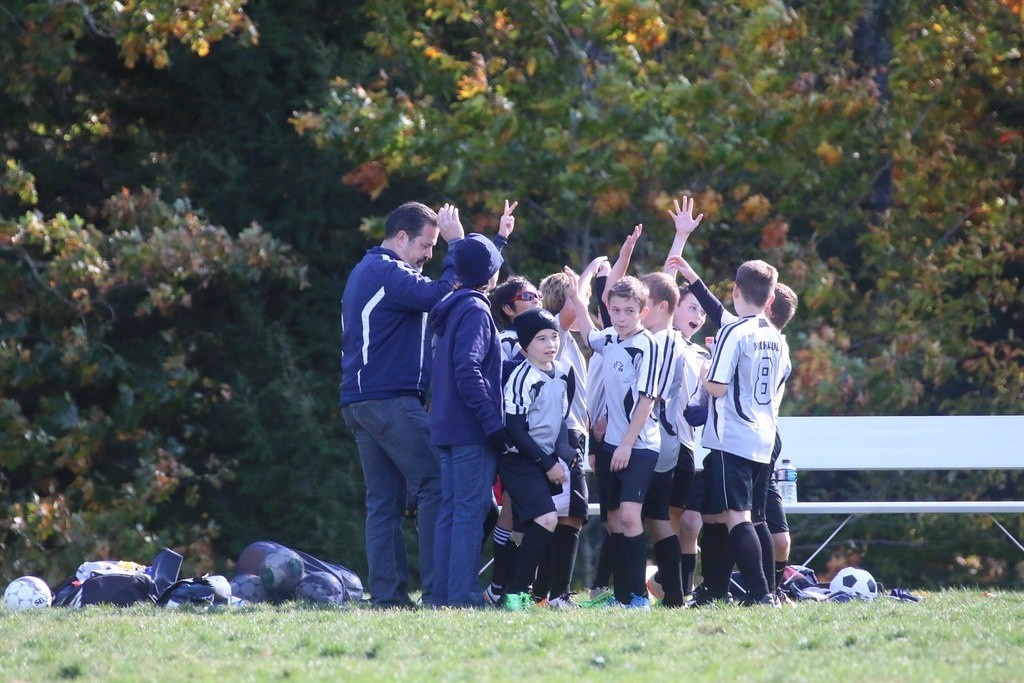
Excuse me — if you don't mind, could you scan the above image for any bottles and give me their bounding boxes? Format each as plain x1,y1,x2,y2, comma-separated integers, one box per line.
778,457,798,504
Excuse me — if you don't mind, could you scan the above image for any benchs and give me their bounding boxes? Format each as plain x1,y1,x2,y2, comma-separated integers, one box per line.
493,412,1022,591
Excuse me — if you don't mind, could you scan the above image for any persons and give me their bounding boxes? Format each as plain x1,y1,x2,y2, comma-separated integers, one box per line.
486,192,800,612
337,201,465,611
426,197,516,607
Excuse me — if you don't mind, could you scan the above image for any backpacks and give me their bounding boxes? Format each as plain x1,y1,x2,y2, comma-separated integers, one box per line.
52,571,159,607
155,573,232,613
149,548,184,592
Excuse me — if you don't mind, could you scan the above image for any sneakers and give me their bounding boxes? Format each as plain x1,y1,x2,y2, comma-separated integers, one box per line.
685,591,734,609
775,587,796,609
630,593,651,611
603,598,631,610
646,572,665,601
547,593,584,609
483,584,502,608
736,591,782,610
579,590,616,608
495,584,537,613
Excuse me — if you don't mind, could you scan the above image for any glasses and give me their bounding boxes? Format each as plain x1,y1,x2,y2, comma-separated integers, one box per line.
507,291,544,304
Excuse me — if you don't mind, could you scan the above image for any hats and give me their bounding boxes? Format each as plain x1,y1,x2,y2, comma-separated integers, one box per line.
514,306,559,349
454,232,505,287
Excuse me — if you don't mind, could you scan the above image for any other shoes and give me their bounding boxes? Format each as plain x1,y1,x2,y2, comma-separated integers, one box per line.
590,586,611,599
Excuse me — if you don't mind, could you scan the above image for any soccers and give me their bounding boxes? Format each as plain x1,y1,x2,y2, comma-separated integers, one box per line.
230,542,363,607
829,566,877,600
4,575,52,610
